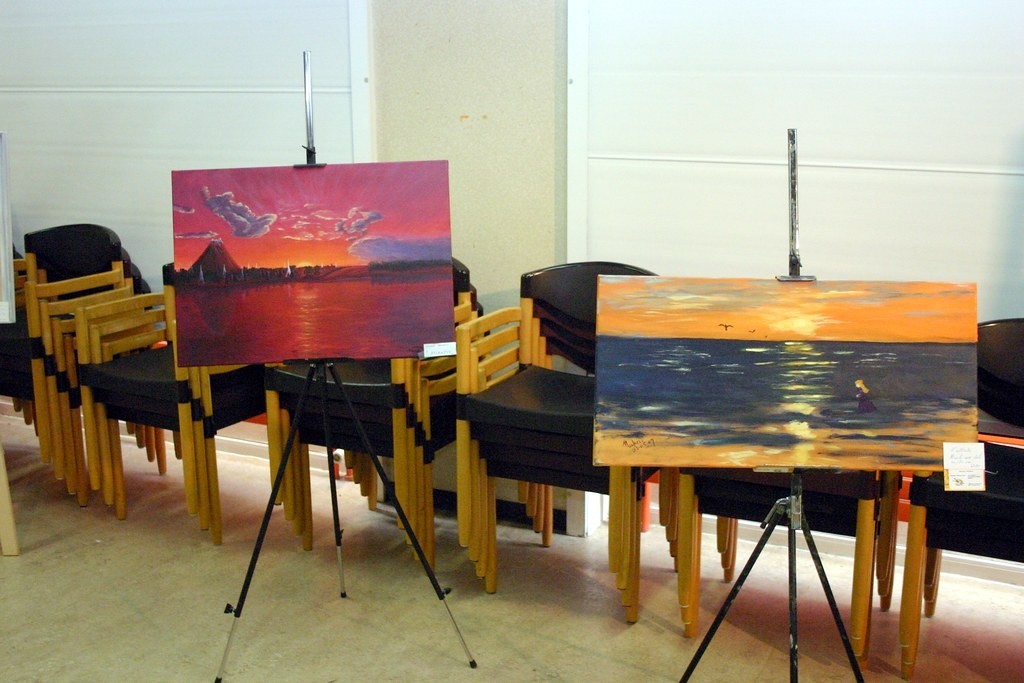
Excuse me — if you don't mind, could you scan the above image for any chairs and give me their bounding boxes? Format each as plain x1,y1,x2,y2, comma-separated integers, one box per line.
0,223,1024,681
